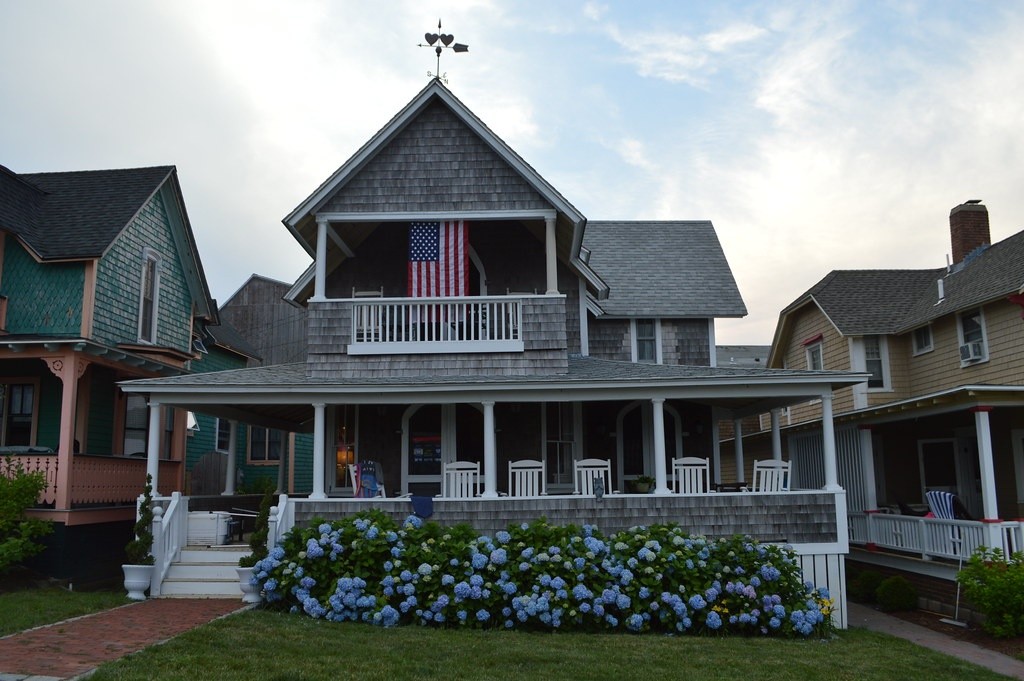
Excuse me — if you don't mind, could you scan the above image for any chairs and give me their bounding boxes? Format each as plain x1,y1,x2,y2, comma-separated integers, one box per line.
752,459,793,491
926,490,973,520
436,461,482,498
500,459,548,496
573,458,621,495
346,463,387,498
672,456,711,493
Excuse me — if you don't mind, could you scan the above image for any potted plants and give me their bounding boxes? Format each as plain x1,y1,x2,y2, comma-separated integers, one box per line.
632,473,656,492
237,485,278,602
121,474,157,601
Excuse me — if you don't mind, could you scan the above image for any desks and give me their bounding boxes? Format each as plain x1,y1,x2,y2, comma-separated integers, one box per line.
704,482,748,492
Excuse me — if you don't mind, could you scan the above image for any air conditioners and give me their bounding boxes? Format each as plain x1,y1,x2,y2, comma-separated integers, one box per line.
959,342,982,362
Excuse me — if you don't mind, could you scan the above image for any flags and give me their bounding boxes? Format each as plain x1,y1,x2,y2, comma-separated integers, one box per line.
406,220,469,324
